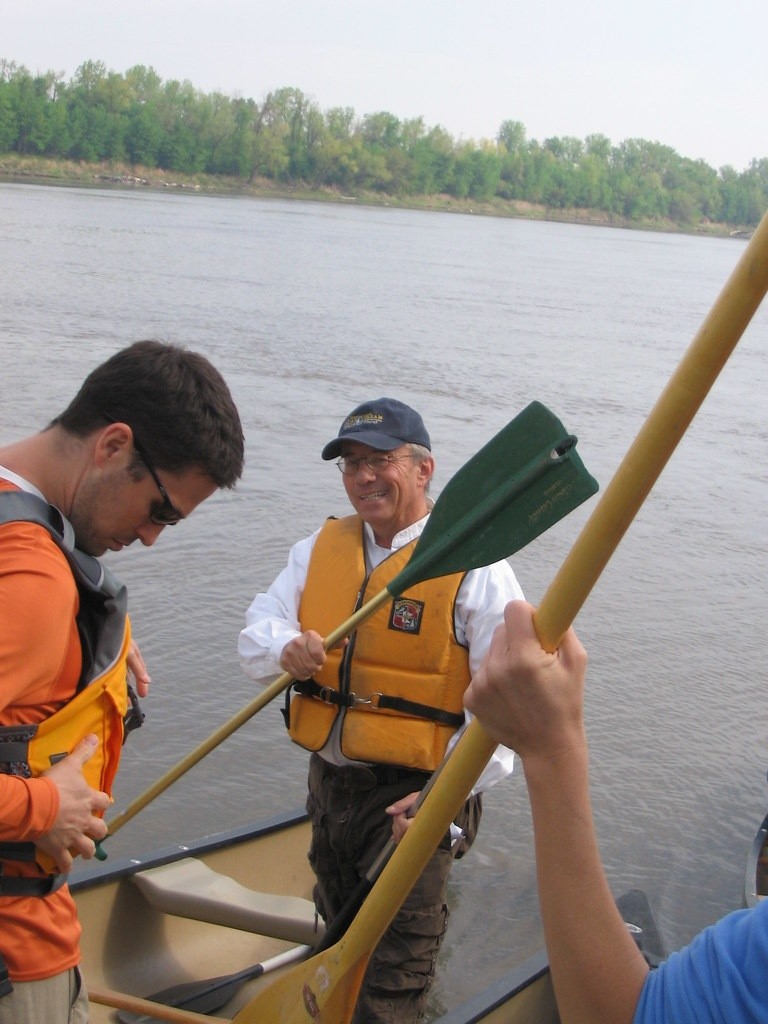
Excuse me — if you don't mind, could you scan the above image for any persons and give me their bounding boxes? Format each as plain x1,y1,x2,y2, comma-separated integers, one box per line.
0,340,245,1024
238,398,526,1024
462,599,767,1024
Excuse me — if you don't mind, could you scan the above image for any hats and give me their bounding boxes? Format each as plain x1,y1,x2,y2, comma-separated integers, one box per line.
321,398,432,460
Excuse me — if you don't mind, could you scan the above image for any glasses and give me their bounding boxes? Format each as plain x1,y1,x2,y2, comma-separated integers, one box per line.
95,410,185,527
336,453,420,474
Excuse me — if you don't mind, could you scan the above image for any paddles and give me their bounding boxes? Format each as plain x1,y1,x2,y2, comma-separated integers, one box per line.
227,211,767,1024
115,942,311,1024
303,726,466,965
95,396,601,849
